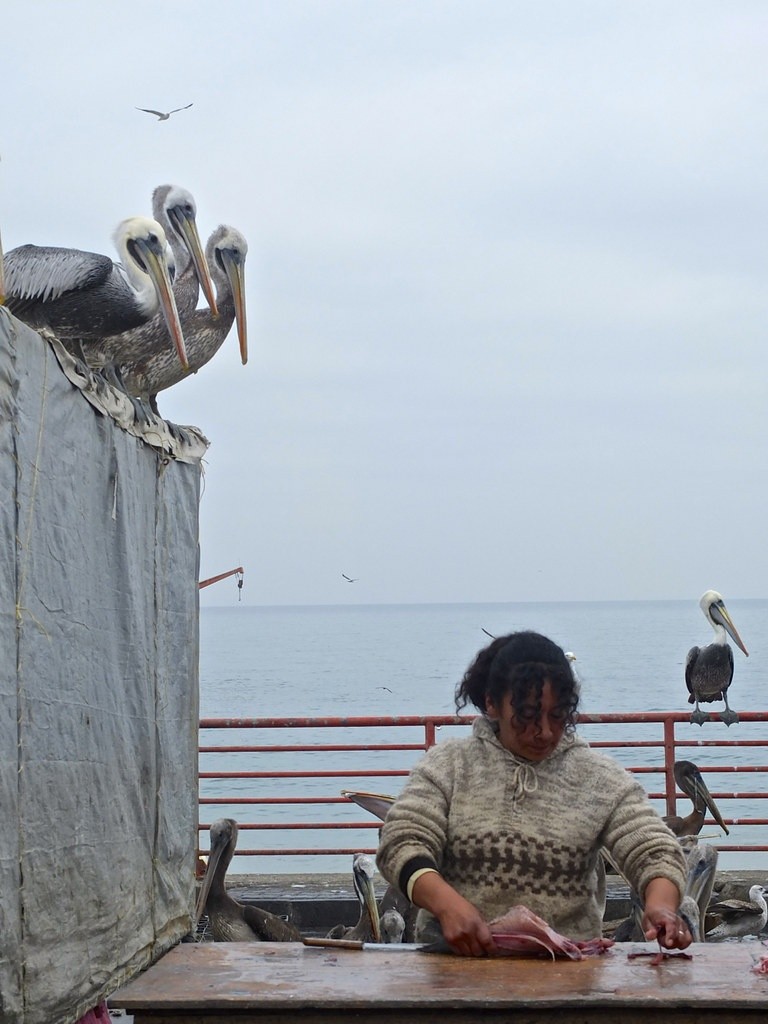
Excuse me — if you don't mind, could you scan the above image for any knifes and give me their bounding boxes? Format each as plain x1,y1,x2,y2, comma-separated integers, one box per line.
301,937,433,951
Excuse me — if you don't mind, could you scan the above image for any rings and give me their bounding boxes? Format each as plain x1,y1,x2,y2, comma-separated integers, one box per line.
678,930,685,935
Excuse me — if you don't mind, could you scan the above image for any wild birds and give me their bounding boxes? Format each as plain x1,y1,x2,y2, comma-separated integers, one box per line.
135,102,194,121
341,573,359,583
608,834,719,943
325,790,421,943
192,819,306,945
376,685,394,693
563,652,583,699
602,760,730,875
685,590,750,727
704,883,768,943
0,183,251,419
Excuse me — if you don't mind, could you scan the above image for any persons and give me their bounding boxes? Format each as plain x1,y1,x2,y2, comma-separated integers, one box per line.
375,630,693,958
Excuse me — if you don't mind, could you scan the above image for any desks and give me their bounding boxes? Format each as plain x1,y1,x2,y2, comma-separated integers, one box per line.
107,942,768,1024
